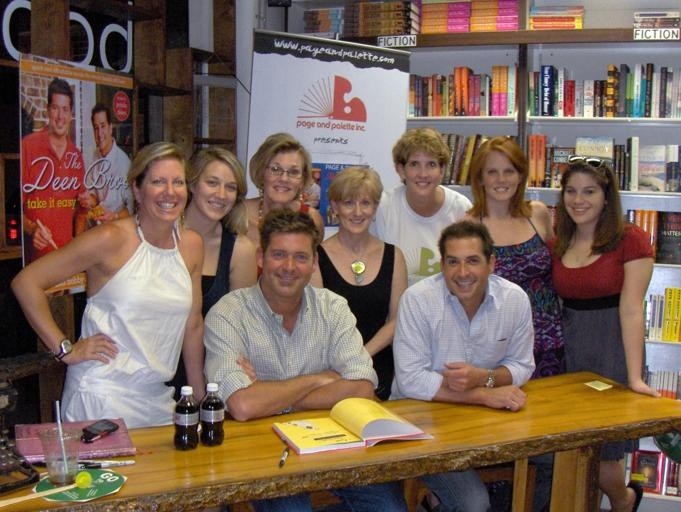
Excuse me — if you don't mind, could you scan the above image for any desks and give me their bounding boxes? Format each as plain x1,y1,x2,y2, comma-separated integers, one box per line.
0,370,680,510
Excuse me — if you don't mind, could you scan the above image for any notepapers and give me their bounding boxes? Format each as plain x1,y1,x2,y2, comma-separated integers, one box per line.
584,380,613,391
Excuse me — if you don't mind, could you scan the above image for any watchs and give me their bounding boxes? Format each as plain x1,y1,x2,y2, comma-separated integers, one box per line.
51,339,72,359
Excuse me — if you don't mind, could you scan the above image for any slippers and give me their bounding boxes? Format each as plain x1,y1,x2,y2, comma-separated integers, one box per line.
610,480,643,512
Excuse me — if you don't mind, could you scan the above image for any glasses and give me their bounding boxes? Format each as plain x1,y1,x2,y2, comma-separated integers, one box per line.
265,166,304,179
567,156,604,169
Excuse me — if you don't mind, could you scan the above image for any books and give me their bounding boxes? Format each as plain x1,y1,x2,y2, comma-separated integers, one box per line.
269,395,438,454
620,209,681,497
633,11,681,29
303,0,584,37
409,62,517,117
529,64,681,119
529,134,681,194
15,417,136,458
441,134,493,184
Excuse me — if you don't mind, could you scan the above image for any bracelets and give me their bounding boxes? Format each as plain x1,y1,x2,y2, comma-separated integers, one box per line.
487,366,496,389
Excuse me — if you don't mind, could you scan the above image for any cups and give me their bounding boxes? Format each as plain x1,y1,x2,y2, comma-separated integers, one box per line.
41,430,79,485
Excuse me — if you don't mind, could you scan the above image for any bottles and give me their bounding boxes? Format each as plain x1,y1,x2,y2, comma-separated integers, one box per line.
202,385,225,443
171,386,199,450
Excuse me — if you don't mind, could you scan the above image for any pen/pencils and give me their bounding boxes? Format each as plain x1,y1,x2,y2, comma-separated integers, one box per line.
77,460,136,470
279,447,290,466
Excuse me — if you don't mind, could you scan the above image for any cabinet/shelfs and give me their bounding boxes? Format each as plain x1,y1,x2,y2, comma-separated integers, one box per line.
338,28,681,500
1,1,237,442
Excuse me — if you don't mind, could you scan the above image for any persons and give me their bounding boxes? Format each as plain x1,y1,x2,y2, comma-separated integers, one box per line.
547,156,664,512
315,167,407,399
390,221,537,512
80,102,137,225
21,77,84,264
461,137,564,381
376,126,473,279
12,141,204,423
204,210,407,511
230,132,323,292
165,146,258,411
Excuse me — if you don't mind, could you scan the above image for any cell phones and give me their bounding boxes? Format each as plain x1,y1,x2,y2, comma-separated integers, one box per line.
80,420,118,443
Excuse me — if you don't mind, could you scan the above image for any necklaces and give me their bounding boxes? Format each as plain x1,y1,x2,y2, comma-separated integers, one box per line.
337,233,368,283
257,197,262,223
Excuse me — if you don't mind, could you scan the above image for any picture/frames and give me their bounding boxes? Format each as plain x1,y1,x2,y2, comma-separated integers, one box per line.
623,445,667,494
